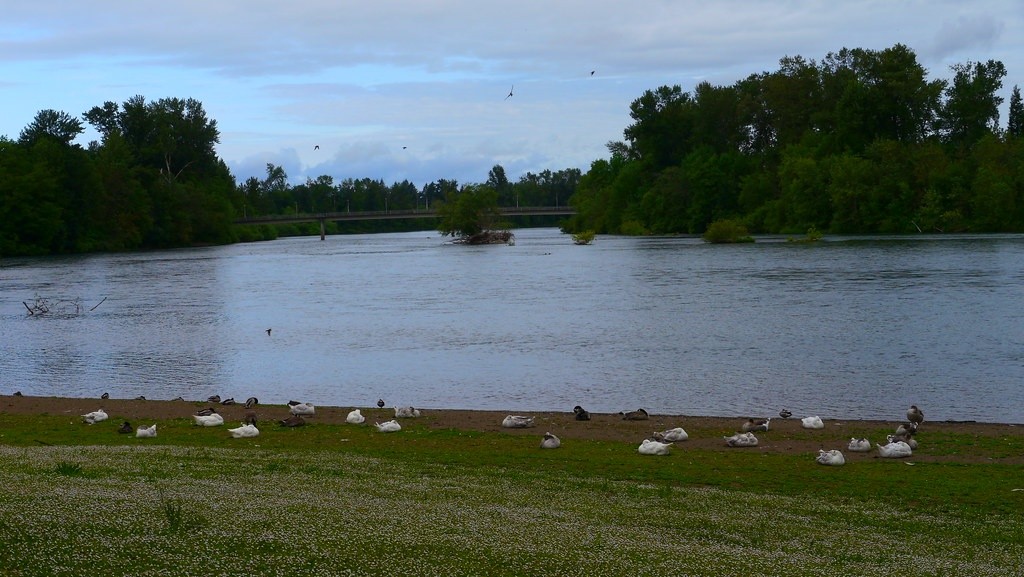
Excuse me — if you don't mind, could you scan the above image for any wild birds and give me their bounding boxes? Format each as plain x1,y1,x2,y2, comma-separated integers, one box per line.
10,390,24,396
78,392,924,467
313,145,320,151
589,69,596,77
265,327,272,336
503,84,515,101
401,146,407,149
